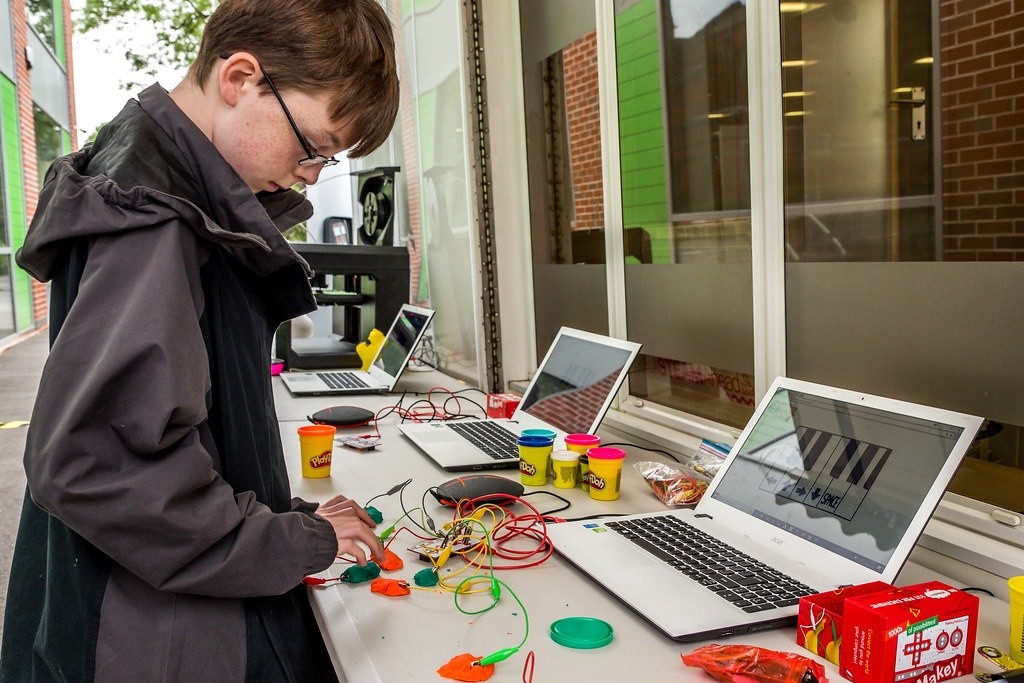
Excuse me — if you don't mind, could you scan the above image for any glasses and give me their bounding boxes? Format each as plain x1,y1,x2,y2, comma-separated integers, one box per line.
220,52,340,169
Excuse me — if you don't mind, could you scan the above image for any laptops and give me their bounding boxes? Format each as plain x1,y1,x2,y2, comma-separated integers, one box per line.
532,376,986,642
279,303,435,396
396,325,645,473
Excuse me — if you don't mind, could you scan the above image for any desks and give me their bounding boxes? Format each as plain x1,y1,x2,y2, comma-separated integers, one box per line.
270,337,1023,683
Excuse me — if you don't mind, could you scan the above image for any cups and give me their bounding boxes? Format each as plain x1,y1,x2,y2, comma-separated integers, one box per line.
1008,576,1024,666
578,455,590,492
586,448,626,500
521,429,556,439
550,451,581,489
298,425,336,479
516,436,554,486
564,433,601,455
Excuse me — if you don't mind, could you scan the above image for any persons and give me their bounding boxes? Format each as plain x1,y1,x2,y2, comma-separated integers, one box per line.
0,0,401,683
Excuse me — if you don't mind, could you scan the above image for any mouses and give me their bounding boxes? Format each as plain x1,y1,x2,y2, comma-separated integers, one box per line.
307,405,375,428
436,475,524,506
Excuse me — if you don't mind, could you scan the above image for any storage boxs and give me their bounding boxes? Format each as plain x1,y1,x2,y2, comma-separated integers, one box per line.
839,581,979,682
797,582,898,667
486,393,522,420
